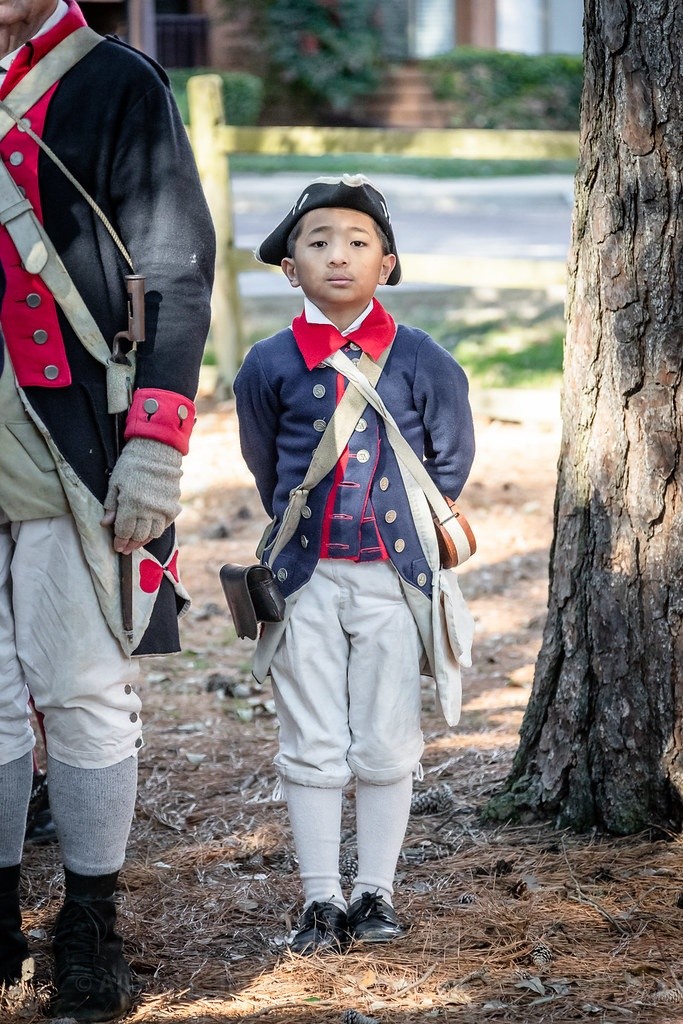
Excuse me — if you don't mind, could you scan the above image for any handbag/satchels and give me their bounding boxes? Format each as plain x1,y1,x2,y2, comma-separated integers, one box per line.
431,570,475,729
219,562,286,643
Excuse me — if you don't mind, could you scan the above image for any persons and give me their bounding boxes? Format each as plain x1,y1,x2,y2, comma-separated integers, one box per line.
234,173,475,956
0,0,217,1020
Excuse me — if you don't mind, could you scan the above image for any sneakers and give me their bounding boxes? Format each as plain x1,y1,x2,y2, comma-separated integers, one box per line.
49,899,133,1023
0,929,29,990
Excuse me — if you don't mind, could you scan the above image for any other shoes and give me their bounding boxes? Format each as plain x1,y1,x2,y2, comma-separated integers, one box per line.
26,769,45,825
25,774,59,844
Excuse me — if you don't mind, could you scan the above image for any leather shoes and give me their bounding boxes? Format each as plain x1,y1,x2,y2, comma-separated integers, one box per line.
346,887,403,944
289,894,349,955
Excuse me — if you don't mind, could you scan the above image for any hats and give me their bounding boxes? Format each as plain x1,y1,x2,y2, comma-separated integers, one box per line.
255,173,402,286
